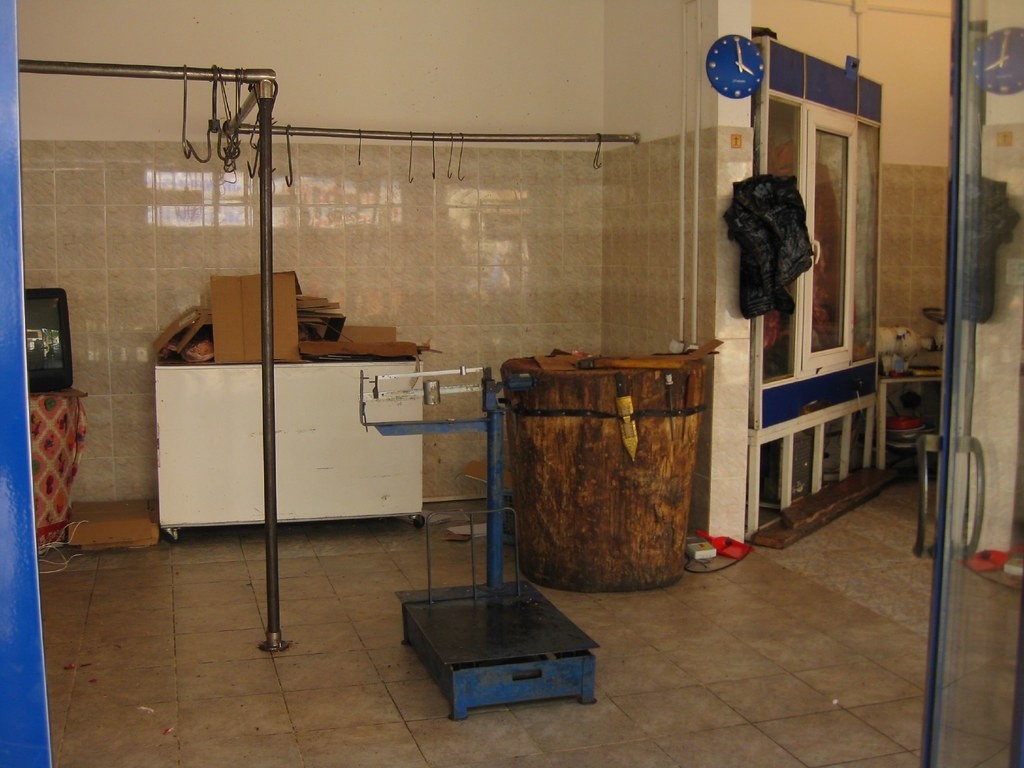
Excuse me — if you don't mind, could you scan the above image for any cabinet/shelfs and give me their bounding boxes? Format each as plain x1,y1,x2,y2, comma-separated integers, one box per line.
747,31,882,528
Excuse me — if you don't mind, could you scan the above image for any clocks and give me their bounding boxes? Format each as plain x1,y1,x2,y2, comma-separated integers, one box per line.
706,35,766,100
970,24,1024,95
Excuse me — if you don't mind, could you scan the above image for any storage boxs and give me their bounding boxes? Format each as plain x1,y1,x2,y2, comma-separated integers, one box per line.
65,498,161,553
209,270,308,362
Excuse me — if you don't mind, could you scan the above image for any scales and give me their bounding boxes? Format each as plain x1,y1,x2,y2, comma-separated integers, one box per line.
360,366,600,721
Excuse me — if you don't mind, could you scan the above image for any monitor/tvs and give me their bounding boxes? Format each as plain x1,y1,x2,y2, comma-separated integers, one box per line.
23,287,73,392
